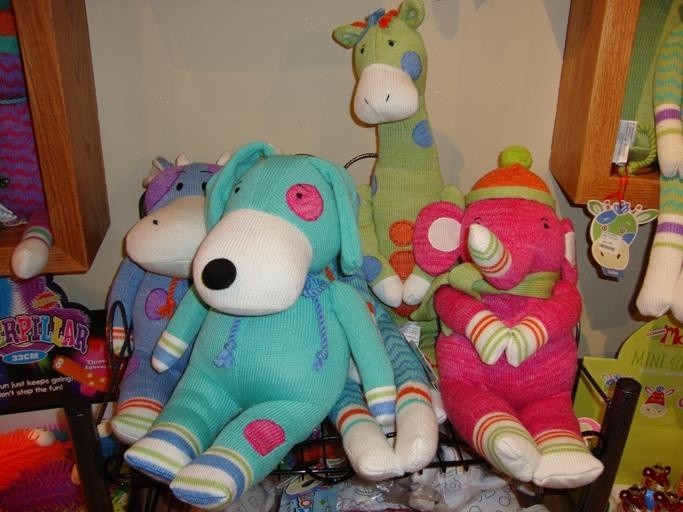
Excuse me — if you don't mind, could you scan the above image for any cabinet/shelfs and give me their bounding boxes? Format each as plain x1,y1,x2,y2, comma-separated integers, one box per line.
64,153,642,512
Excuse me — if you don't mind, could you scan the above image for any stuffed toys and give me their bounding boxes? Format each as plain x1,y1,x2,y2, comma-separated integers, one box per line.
616,463,682,512
104,152,232,445
0,409,132,512
324,256,438,481
122,142,396,512
635,21,682,324
412,146,603,489
0,0,53,280
332,0,465,426
